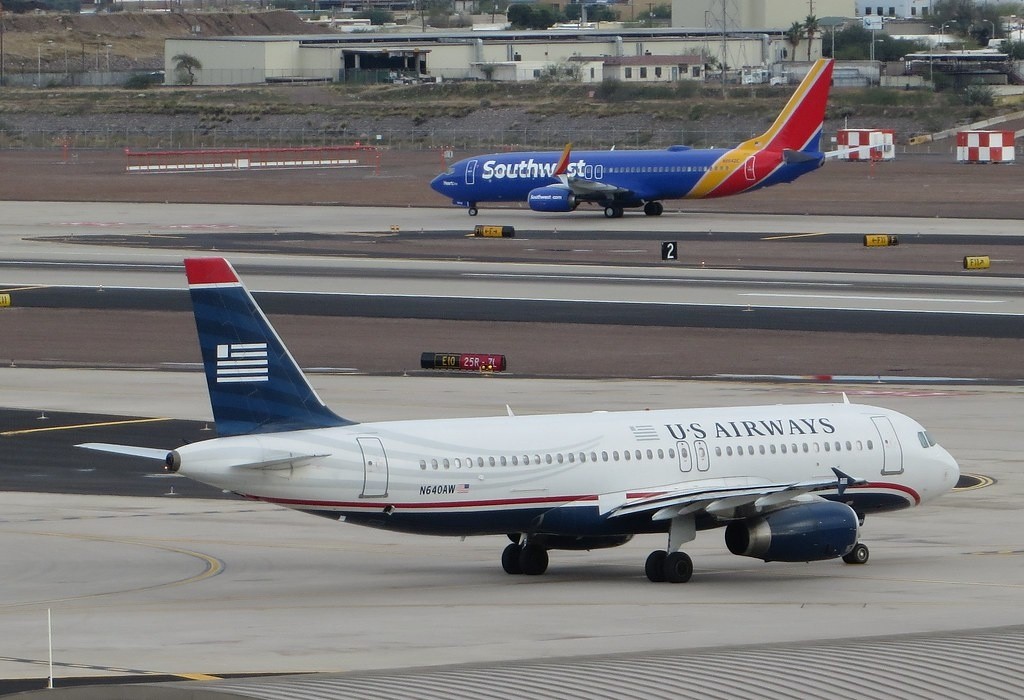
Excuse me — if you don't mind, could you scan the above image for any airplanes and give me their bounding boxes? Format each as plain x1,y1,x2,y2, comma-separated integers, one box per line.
427,60,896,216
71,255,1024,584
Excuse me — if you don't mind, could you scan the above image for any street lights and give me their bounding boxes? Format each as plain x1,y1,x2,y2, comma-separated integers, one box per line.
931,25,949,49
831,21,849,61
870,38,884,87
983,20,995,49
941,20,958,50
